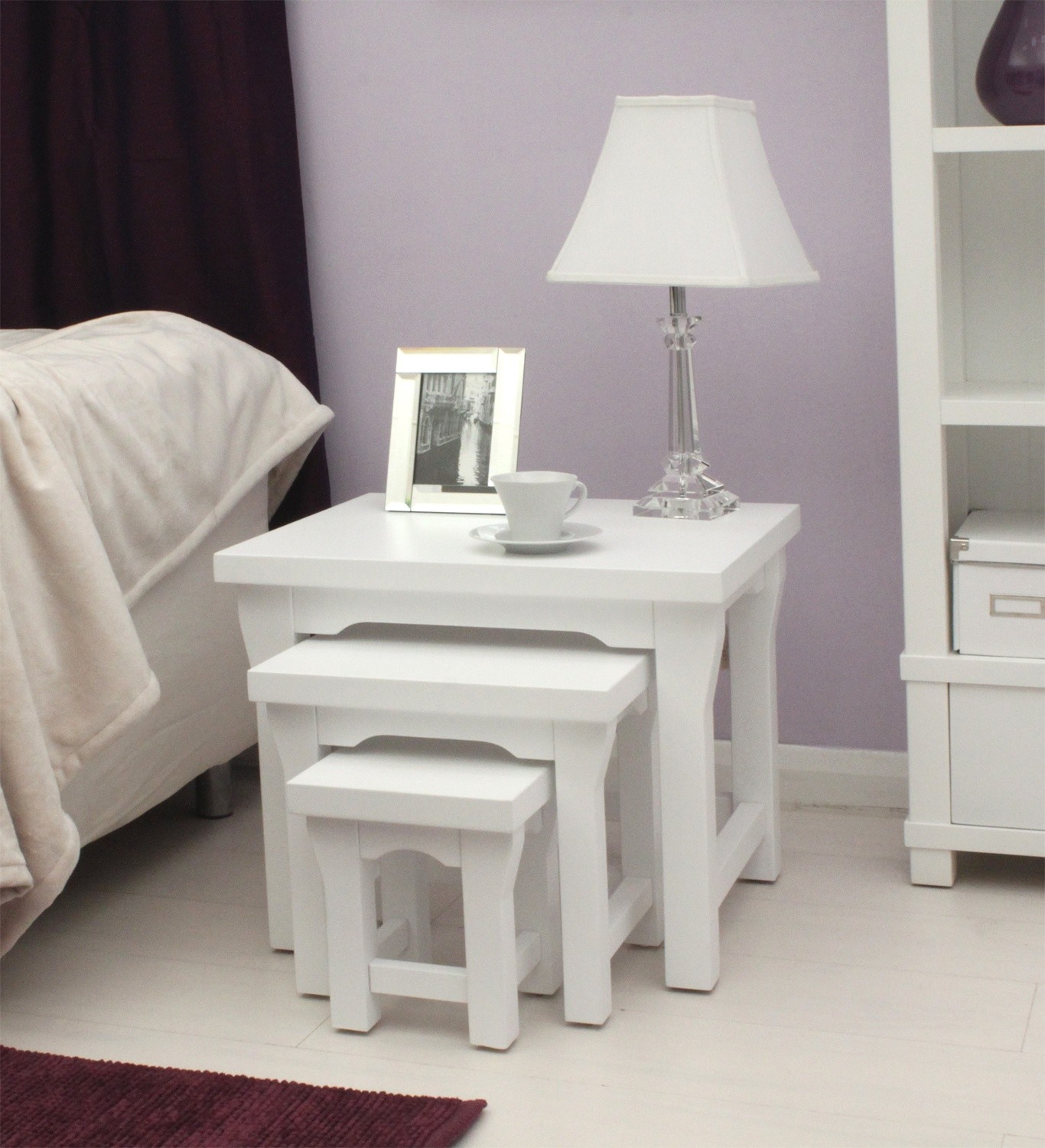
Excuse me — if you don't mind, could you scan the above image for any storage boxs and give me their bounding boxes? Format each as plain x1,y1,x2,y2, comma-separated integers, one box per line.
947,509,1045,659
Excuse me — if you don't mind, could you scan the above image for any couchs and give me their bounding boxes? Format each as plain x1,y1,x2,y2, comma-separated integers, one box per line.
0,307,336,955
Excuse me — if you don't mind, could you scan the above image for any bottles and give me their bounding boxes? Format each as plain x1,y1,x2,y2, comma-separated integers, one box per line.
975,0,1045,126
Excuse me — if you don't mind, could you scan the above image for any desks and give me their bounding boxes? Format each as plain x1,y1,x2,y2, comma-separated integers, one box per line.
215,469,798,993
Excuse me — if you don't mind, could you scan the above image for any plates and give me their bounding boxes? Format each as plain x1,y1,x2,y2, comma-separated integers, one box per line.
469,522,603,554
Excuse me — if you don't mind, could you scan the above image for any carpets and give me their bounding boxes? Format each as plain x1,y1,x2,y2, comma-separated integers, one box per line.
0,1045,488,1148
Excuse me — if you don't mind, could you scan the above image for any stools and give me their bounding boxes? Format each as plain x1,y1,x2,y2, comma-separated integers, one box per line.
287,747,564,1052
246,617,668,1026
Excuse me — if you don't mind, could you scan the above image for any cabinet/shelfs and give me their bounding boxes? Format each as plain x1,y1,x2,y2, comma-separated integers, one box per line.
884,1,1045,886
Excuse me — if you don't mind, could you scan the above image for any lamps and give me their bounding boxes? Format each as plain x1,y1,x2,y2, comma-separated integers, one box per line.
546,93,819,517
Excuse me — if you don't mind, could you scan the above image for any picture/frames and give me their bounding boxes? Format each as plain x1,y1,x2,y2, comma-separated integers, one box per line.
387,349,525,518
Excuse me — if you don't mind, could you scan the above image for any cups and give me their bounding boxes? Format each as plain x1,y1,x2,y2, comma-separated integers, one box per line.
491,471,587,541
